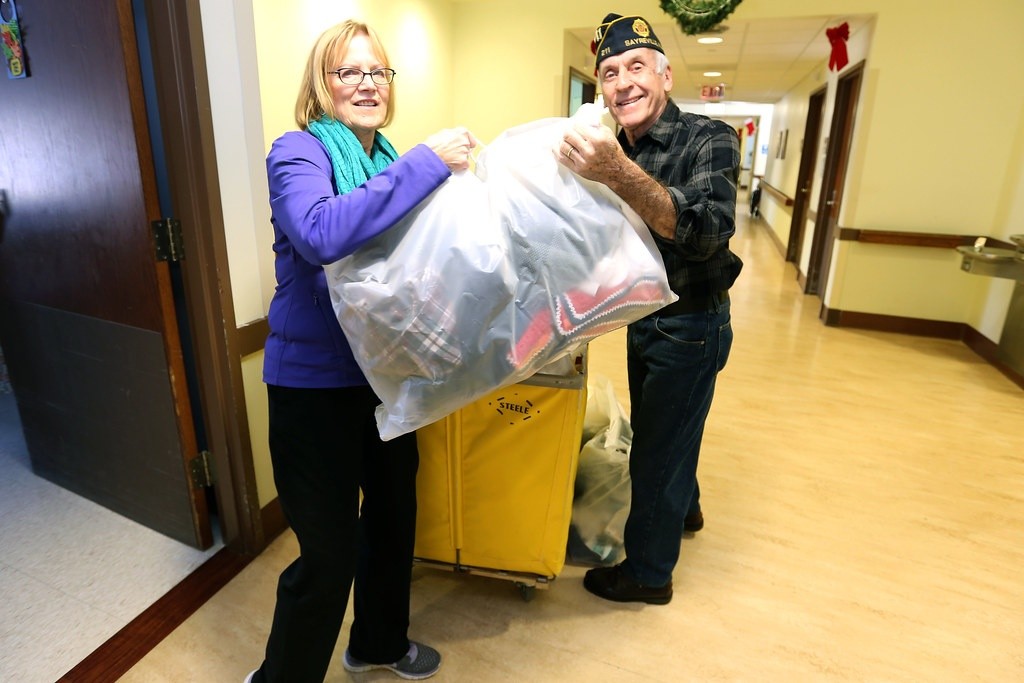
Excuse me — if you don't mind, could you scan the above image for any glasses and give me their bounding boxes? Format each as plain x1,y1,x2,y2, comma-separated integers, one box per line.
327,68,397,85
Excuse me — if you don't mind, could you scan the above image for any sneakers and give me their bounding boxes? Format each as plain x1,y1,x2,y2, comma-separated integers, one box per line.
683,512,703,531
584,559,674,605
343,639,441,680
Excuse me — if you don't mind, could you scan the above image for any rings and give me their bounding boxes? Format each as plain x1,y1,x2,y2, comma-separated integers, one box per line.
567,146,575,158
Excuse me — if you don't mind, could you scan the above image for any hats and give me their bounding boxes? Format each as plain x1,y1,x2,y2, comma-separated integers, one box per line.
590,12,666,76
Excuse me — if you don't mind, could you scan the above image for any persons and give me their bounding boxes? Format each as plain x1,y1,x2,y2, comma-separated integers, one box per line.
558,12,743,606
242,19,477,683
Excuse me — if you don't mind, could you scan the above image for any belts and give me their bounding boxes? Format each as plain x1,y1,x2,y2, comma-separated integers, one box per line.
655,291,729,318
